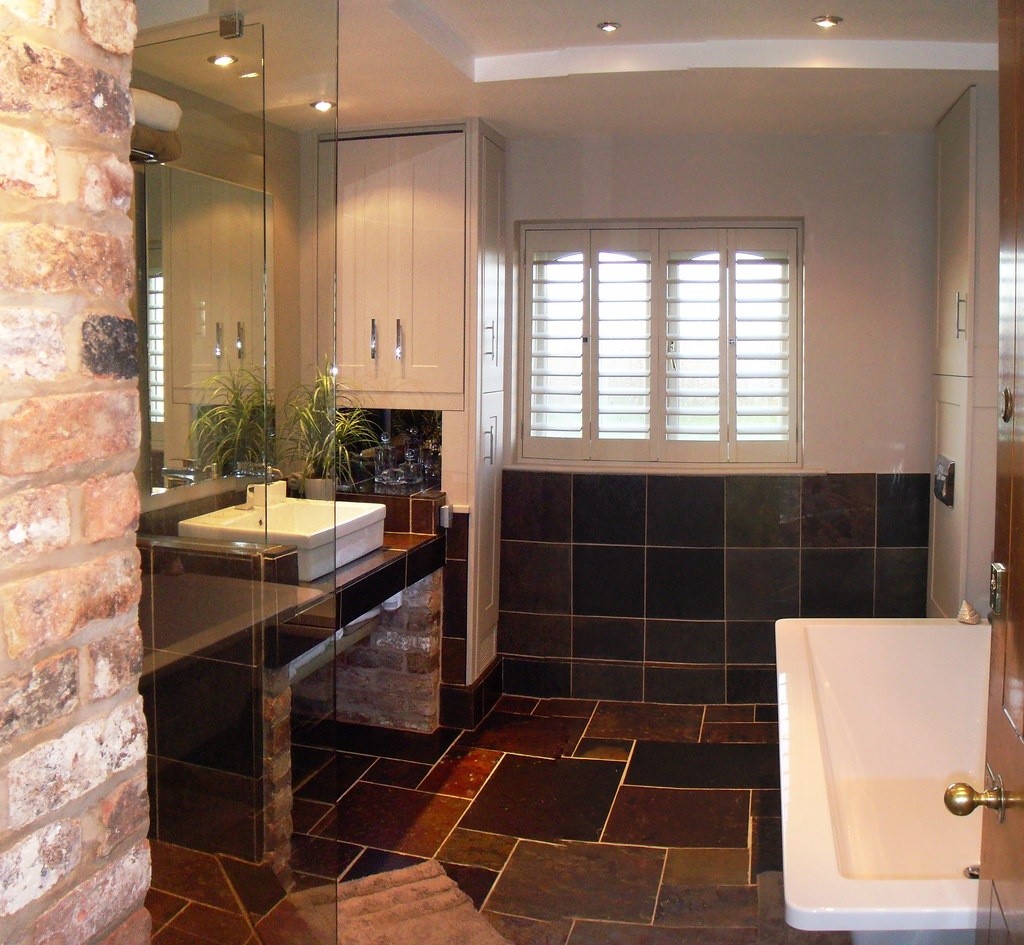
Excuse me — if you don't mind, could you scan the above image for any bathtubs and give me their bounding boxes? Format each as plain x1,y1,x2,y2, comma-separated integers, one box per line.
768,610,998,945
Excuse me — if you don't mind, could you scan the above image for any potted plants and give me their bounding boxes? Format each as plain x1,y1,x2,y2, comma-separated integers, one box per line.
183,363,277,474
280,354,384,501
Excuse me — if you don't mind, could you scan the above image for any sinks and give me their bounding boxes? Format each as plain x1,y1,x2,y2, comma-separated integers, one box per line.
178,496,387,583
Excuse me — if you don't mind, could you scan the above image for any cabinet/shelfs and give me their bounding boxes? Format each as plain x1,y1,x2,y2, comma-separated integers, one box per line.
298,116,507,412
161,167,273,410
438,391,505,685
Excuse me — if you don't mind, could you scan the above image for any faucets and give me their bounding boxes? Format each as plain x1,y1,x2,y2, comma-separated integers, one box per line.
159,458,221,481
237,461,284,484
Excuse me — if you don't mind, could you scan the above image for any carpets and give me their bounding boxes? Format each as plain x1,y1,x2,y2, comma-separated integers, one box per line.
254,859,514,945
